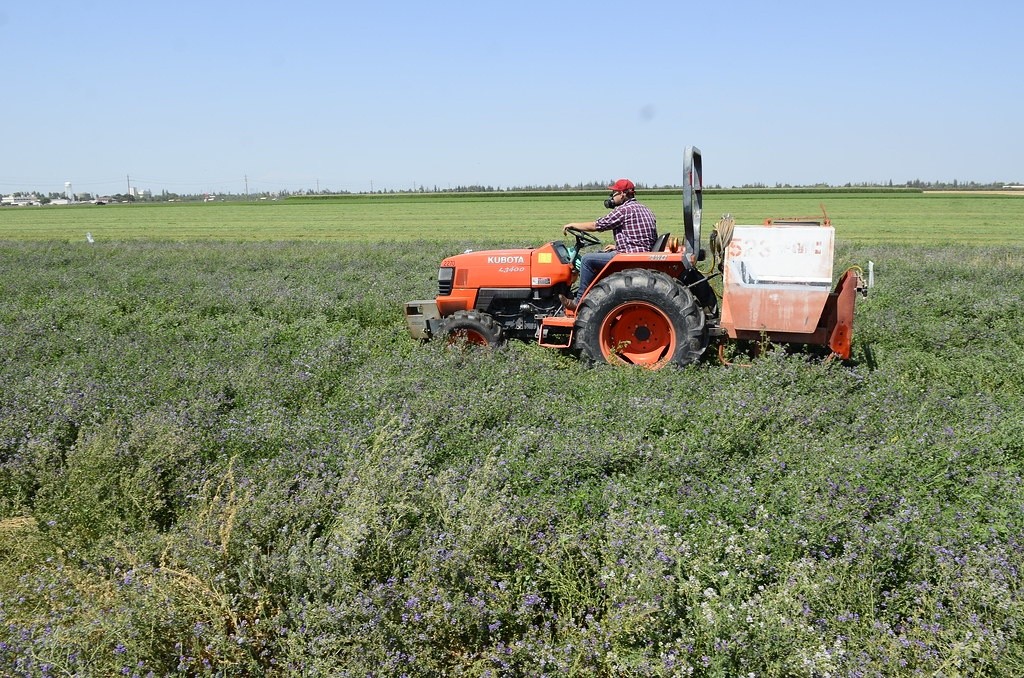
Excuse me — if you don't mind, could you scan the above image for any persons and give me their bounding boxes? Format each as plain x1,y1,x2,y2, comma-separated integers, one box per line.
559,179,657,311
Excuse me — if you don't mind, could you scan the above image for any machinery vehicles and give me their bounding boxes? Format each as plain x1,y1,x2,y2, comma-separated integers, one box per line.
402,146,868,375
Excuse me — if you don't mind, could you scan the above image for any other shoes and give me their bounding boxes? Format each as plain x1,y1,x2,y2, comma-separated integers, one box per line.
559,294,576,312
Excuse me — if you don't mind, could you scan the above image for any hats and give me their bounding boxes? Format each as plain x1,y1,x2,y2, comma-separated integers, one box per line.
608,179,635,193
